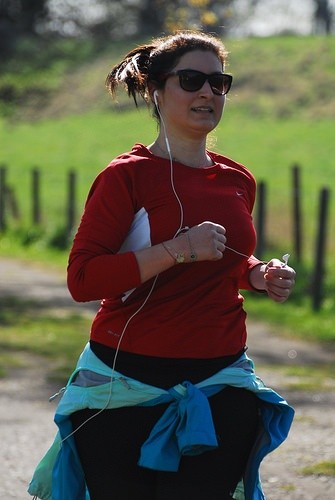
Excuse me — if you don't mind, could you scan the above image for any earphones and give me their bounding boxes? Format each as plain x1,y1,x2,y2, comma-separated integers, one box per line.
153,90,159,105
281,254,290,268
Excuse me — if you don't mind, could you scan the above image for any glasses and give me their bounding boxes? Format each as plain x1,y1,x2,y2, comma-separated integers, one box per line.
157,68,233,96
